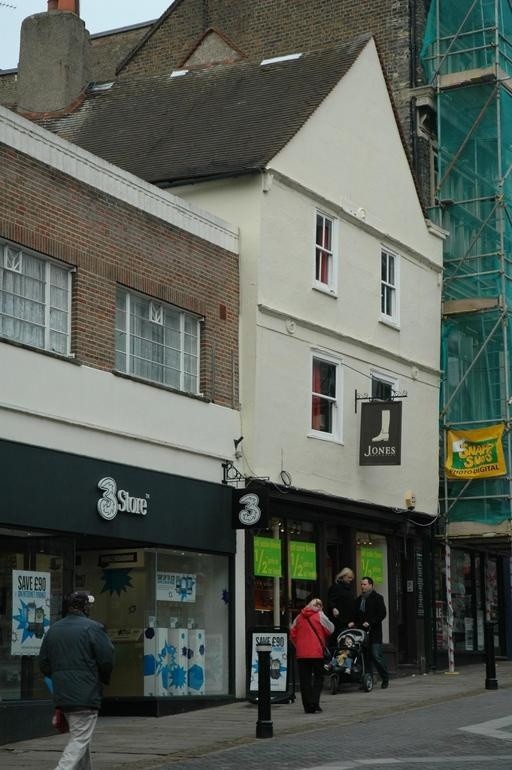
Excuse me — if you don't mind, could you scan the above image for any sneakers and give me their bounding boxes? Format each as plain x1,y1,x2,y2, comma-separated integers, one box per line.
381,681,388,688
345,668,351,675
323,664,330,672
313,703,322,711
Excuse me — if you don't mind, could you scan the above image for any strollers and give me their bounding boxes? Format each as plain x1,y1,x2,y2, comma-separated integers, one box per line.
324,627,373,695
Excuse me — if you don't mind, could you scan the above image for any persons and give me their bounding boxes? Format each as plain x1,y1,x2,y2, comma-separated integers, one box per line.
347,577,389,689
323,633,355,675
289,597,335,713
39,591,115,770
328,567,356,647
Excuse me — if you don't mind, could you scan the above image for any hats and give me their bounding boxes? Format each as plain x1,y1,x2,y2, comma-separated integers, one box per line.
66,590,94,605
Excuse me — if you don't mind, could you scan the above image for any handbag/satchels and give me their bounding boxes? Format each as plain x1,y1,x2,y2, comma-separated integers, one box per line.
51,708,69,734
323,648,332,665
